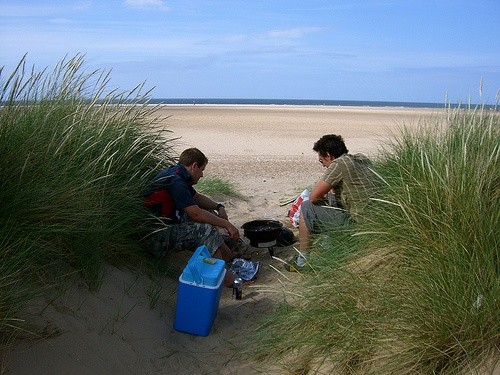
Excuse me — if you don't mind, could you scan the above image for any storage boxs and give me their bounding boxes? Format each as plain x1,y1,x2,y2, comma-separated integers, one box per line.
174,245,228,337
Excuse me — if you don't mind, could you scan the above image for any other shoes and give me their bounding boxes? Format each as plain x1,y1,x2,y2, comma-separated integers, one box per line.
284,262,306,272
226,254,251,262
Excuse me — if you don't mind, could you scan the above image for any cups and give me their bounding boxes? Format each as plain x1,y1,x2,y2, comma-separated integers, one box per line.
232,278,243,300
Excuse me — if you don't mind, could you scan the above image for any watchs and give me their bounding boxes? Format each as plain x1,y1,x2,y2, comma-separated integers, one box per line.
216,203,225,212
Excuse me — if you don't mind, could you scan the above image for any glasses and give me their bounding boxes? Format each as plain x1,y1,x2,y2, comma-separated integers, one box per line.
320,158,324,163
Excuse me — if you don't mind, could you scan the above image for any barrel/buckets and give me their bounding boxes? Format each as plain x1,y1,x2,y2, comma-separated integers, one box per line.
173,244,228,337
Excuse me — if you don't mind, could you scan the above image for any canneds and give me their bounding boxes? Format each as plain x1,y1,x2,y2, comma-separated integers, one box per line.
232,278,242,301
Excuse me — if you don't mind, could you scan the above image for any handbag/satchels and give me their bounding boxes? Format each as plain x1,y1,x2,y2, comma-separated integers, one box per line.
290,189,309,228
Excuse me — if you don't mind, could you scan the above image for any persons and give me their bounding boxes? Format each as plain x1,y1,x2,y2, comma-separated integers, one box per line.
285,133,380,272
134,147,260,281
